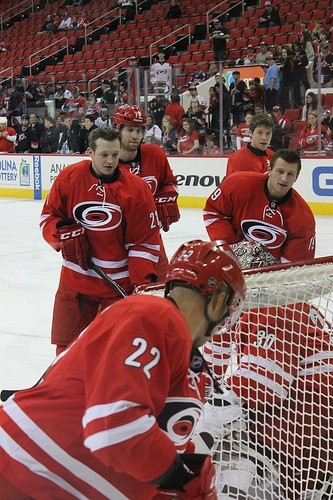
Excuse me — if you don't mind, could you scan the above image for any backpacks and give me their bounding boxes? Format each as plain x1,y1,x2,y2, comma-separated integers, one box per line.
25,84,41,102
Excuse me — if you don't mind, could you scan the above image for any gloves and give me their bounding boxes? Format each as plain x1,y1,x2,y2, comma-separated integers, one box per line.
153,191,180,232
131,278,152,295
228,238,254,270
52,222,92,272
153,451,219,500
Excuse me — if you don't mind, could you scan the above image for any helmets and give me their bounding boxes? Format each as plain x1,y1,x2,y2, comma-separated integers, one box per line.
113,105,147,128
0,117,8,124
163,238,245,308
21,114,30,120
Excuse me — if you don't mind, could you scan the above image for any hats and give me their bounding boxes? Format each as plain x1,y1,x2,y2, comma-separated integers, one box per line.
271,105,280,113
100,78,126,87
95,98,104,104
128,55,138,61
247,44,253,49
309,110,318,118
213,18,221,24
215,73,225,82
258,42,266,45
319,41,329,48
158,49,164,53
266,56,273,60
55,85,63,91
232,70,240,75
188,85,196,91
71,86,81,93
264,1,272,7
101,107,109,114
153,56,160,61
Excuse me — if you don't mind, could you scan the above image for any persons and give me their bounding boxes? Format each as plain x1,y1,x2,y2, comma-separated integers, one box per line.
115,104,180,232
203,272,333,500
0,0,333,155
203,149,315,263
0,238,247,500
226,113,276,176
40,127,170,357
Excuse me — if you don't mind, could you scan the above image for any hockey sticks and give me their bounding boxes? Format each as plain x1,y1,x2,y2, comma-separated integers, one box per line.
88,259,128,300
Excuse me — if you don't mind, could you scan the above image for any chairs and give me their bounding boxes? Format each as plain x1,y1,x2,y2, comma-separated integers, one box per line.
0,0,333,139
